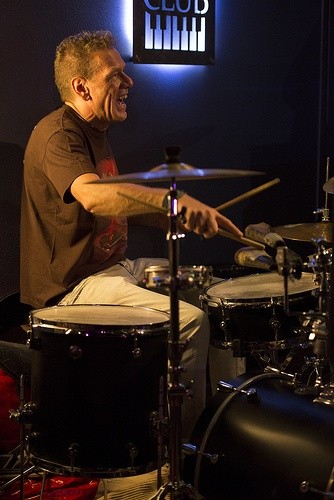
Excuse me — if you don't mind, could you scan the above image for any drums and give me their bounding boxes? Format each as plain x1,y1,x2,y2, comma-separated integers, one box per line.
144,265,207,289
203,270,326,353
22,304,172,479
179,366,334,500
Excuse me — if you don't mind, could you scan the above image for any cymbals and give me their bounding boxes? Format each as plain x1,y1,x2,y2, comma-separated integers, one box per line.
84,163,271,184
323,177,334,194
271,222,333,242
